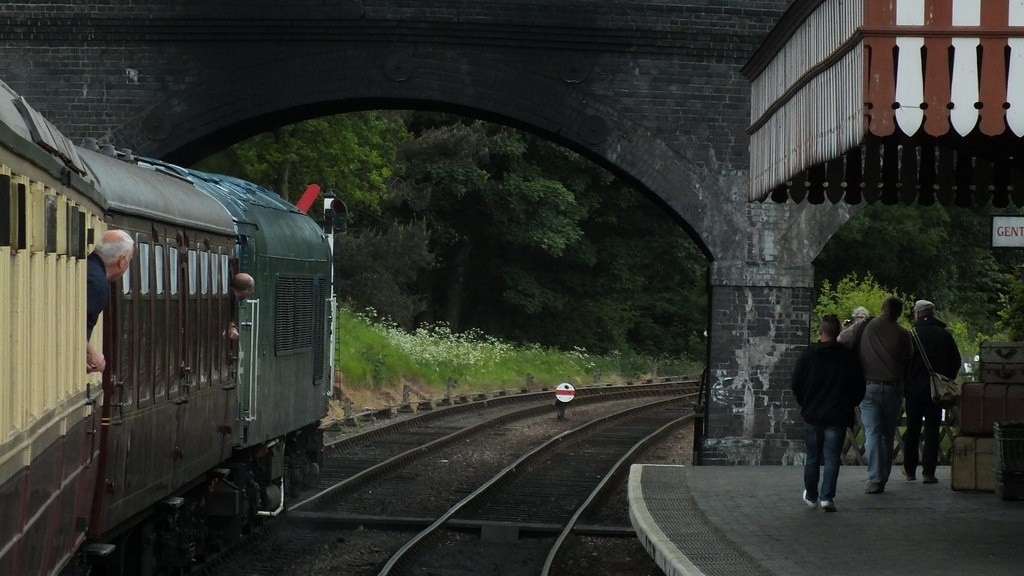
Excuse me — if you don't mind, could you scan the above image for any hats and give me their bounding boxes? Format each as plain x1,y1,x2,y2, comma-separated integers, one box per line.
914,299,935,315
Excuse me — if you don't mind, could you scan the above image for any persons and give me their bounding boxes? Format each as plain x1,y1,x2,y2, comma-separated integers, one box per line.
230,273,255,341
902,299,962,483
837,298,915,494
85,229,131,375
841,307,870,330
792,315,868,513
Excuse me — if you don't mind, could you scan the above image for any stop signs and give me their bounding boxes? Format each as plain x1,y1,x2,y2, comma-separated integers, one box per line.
556,384,576,402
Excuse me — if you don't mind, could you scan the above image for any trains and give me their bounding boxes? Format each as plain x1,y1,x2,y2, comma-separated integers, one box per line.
0,78,348,576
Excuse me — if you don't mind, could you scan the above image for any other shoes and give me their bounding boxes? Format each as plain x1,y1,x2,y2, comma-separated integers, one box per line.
923,475,937,483
865,488,880,494
902,468,915,481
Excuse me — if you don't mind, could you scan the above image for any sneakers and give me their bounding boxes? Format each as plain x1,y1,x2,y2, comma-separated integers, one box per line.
821,501,836,512
803,489,817,508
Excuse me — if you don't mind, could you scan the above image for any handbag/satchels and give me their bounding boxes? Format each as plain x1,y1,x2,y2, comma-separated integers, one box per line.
930,372,961,409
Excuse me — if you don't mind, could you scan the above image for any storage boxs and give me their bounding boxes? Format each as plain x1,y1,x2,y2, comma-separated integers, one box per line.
994,472,1024,500
993,419,1024,472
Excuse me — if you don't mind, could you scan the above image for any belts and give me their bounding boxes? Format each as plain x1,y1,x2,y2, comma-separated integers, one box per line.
868,380,895,385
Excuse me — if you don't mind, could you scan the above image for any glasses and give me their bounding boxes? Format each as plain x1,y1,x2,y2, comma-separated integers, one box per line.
823,314,838,320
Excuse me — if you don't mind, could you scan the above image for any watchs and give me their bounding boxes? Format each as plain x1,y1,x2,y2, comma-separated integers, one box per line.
232,325,238,330
